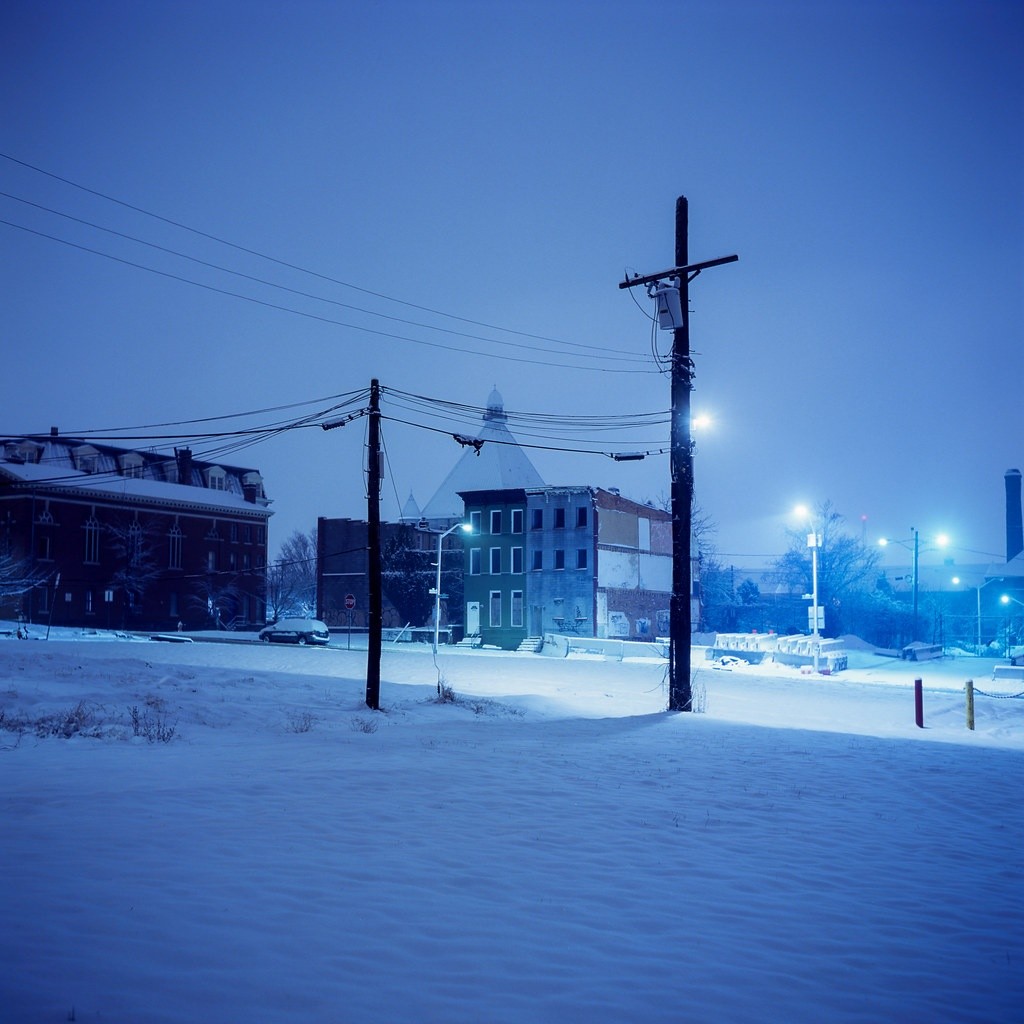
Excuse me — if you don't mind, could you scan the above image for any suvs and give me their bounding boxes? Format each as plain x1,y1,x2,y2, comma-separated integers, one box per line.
259,620,329,645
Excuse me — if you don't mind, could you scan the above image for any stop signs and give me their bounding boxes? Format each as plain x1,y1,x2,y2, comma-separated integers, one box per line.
345,595,355,610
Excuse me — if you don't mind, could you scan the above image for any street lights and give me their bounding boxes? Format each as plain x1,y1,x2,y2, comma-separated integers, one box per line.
434,523,472,652
794,505,818,633
952,574,1004,656
